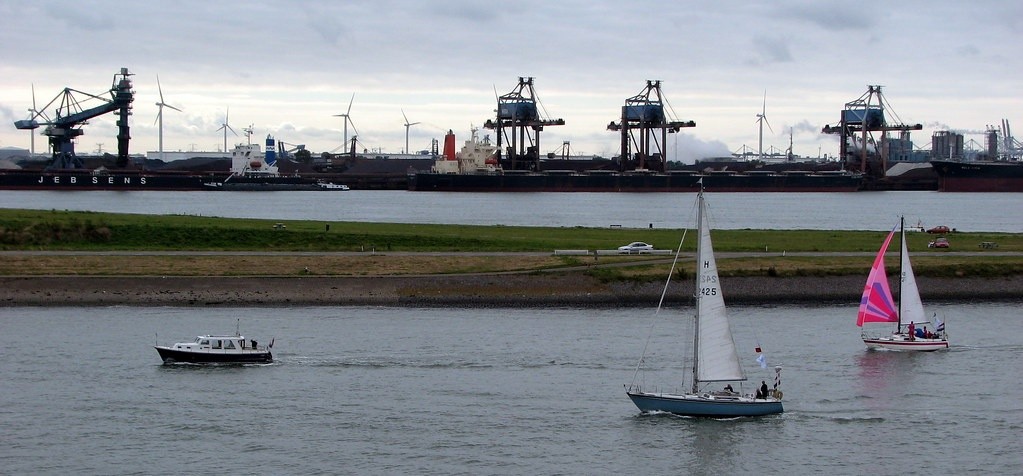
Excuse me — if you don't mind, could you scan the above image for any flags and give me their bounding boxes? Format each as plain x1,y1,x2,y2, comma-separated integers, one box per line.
755,347,761,353
756,354,766,369
937,323,944,331
269,338,274,348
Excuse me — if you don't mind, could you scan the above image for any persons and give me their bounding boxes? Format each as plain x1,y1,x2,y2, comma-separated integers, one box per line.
761,380,768,396
908,321,915,342
756,389,762,397
924,326,937,339
228,340,234,347
931,239,935,244
724,384,733,392
251,340,258,349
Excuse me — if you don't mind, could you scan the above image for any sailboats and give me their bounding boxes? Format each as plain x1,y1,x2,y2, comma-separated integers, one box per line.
620,179,787,415
856,211,952,352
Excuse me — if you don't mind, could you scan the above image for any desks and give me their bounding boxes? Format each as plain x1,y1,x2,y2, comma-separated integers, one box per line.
981,242,996,249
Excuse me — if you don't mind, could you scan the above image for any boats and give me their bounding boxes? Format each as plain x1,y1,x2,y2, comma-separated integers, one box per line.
153,318,275,364
197,125,350,191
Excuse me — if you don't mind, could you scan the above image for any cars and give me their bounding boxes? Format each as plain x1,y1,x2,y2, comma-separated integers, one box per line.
618,241,653,253
927,225,951,234
934,237,949,248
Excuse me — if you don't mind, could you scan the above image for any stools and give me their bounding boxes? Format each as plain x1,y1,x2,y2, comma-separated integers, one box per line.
978,244,981,247
996,244,999,248
